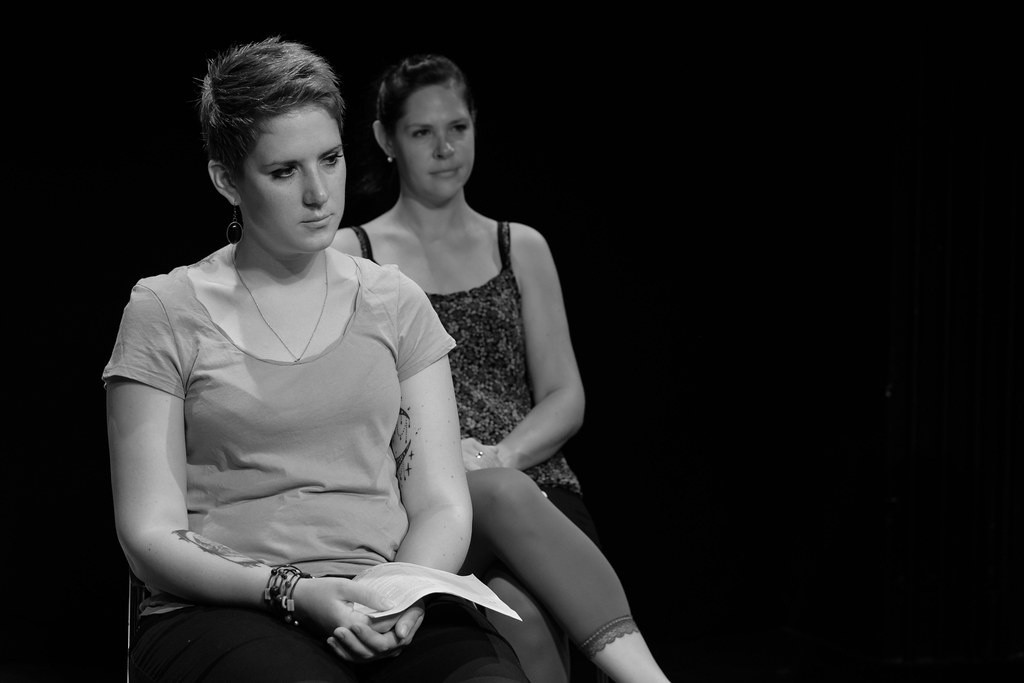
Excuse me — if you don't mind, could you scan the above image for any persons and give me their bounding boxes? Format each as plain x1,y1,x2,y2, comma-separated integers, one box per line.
326,54,671,683
104,37,530,683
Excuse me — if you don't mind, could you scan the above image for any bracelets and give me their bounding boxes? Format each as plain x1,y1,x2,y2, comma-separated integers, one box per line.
266,565,315,629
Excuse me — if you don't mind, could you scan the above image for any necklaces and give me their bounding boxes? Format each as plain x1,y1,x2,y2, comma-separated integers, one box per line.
233,242,329,362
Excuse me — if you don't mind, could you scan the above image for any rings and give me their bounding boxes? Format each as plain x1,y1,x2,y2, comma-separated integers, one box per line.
476,451,485,459
